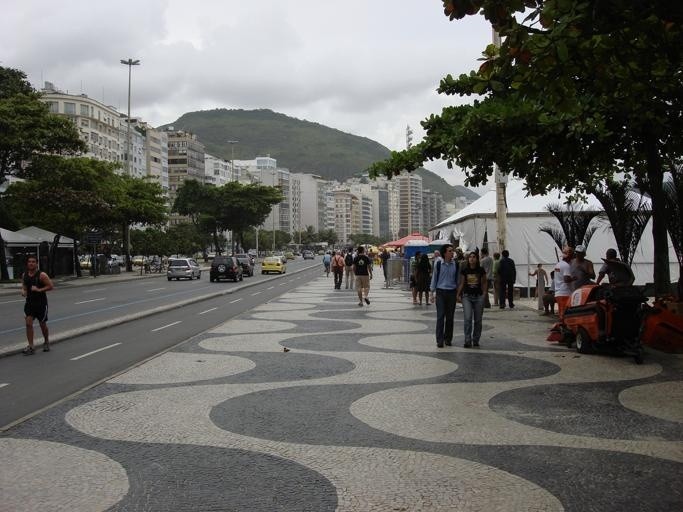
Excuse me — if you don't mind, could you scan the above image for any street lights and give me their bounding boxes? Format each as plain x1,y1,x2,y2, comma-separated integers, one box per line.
118,57,142,177
226,140,238,181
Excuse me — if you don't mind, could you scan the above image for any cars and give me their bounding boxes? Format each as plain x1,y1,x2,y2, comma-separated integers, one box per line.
71,248,163,274
259,256,287,276
233,251,253,274
166,257,201,281
271,248,332,261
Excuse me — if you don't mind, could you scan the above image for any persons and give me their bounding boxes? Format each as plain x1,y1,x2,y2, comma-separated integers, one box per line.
322,244,516,348
527,245,635,321
21,256,53,355
110,256,162,271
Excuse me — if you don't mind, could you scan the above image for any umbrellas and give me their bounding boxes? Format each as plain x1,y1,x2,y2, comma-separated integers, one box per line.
384,234,431,246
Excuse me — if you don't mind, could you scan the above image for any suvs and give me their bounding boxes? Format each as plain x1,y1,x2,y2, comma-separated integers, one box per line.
209,254,243,282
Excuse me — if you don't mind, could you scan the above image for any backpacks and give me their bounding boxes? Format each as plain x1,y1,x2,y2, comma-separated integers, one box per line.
345,253,353,266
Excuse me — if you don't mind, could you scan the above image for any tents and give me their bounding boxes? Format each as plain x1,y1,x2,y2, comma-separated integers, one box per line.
427,180,681,286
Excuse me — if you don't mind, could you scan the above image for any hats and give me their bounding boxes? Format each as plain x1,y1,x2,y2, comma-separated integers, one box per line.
575,245,586,254
560,247,573,258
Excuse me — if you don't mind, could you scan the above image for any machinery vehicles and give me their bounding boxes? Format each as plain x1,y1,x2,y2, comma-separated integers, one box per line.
547,282,683,363
